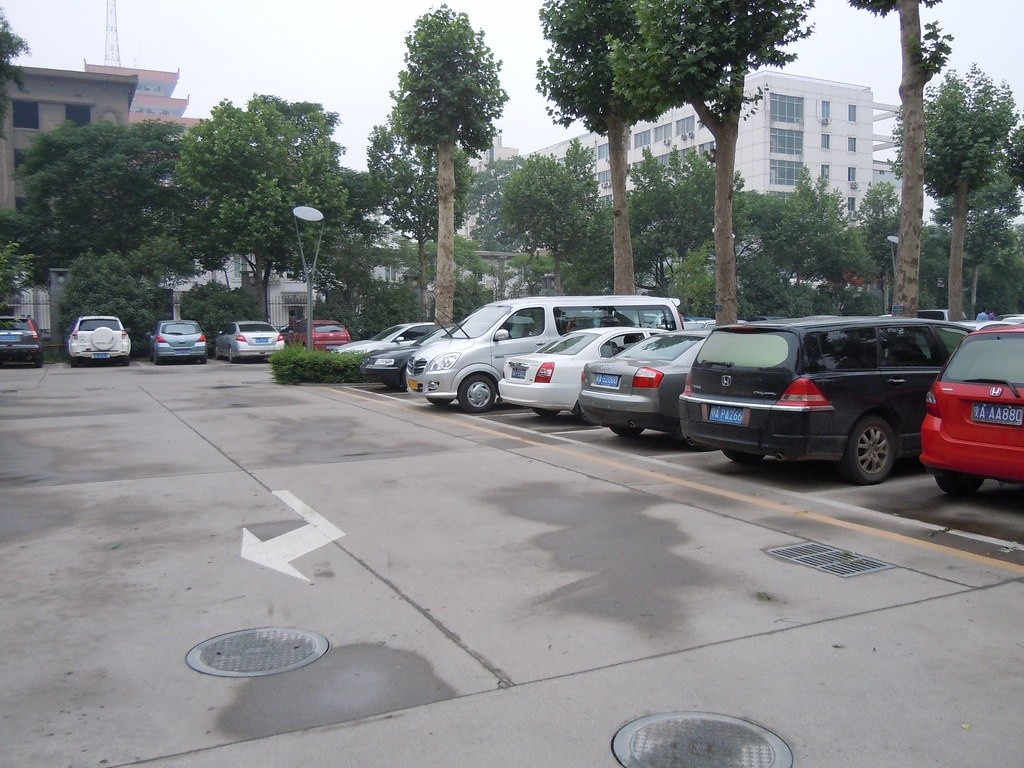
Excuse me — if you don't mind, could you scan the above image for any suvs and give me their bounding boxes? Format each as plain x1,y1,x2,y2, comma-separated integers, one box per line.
68,314,132,368
0,313,46,368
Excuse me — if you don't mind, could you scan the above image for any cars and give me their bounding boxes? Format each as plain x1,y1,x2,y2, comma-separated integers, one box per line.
215,319,288,364
919,322,1024,496
577,330,721,451
329,321,435,389
287,319,352,354
881,309,1024,330
495,326,670,426
360,323,460,393
677,314,978,486
643,312,749,331
145,319,209,365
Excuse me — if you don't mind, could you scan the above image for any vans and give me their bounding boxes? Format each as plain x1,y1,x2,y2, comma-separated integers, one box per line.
404,295,687,413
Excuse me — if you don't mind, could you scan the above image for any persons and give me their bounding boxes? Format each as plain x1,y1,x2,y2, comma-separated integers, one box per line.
976,307,996,321
290,311,296,327
